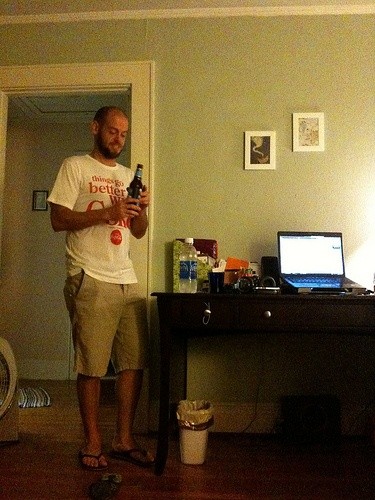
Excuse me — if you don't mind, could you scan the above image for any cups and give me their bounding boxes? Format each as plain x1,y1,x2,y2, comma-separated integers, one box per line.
208,272,224,293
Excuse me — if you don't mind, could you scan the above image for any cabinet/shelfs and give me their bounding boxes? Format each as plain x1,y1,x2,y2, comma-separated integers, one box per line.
149,287,375,477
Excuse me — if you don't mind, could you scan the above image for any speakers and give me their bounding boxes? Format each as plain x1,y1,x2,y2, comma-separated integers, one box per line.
260,257,281,288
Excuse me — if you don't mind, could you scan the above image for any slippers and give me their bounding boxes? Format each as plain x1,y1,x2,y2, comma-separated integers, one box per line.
77,450,108,473
111,448,156,467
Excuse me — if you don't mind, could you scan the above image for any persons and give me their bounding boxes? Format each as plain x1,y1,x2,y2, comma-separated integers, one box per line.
47,106,156,472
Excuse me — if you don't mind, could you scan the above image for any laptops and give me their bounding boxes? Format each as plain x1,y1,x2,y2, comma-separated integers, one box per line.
277,231,367,293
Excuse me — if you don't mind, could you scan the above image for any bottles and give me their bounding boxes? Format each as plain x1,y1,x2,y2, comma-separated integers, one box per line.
179,238,197,293
238,267,260,293
128,164,144,214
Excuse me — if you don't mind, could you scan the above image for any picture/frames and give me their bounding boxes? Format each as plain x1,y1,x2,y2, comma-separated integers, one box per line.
244,130,277,170
291,111,325,152
32,190,49,212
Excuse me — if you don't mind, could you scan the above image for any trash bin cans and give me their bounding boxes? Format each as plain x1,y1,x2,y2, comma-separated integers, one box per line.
177,399,214,465
